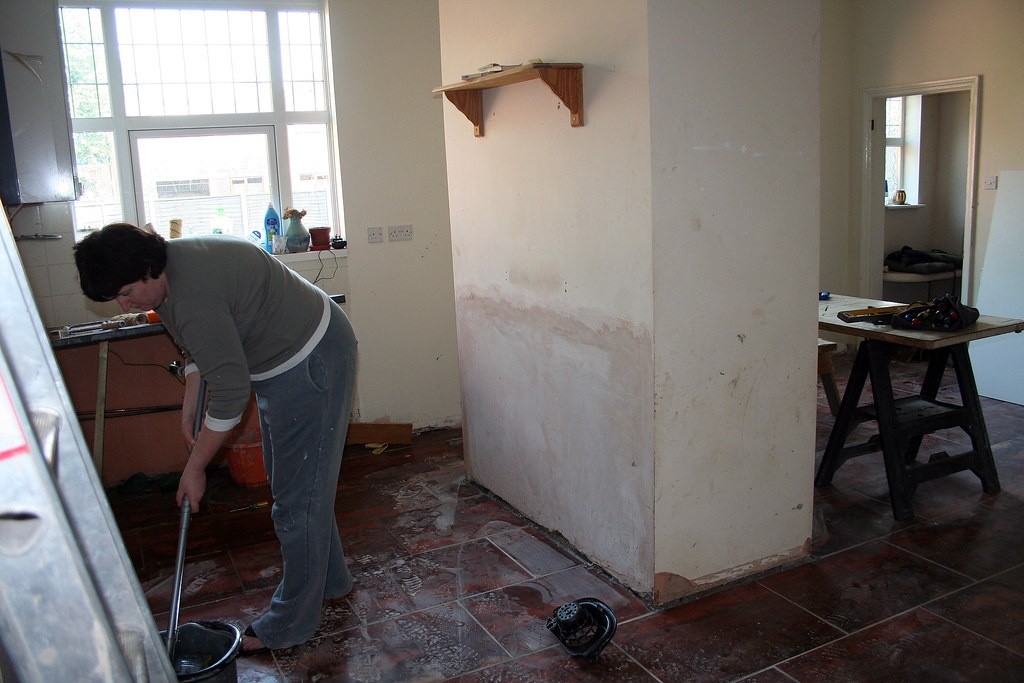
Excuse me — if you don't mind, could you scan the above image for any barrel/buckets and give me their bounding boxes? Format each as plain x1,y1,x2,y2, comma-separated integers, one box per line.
222,420,267,488
159,619,243,683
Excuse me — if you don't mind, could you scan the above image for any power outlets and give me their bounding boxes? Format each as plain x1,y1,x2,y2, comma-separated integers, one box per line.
387,225,413,241
367,227,384,243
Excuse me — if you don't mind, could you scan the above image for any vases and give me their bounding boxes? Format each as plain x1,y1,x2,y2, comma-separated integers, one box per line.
284,217,309,253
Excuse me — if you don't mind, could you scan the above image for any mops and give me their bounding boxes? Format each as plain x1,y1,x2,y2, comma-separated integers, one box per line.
165,380,214,675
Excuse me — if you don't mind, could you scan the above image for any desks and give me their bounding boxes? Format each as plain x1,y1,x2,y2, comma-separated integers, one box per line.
884,271,962,302
48,295,344,483
818,294,1024,523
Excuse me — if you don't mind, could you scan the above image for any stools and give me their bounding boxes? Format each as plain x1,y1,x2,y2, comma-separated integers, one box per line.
818,338,843,416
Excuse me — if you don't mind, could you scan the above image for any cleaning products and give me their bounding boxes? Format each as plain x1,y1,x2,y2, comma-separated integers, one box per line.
264,202,280,254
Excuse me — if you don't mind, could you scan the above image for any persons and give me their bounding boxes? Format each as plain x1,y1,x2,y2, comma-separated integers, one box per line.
74,223,357,650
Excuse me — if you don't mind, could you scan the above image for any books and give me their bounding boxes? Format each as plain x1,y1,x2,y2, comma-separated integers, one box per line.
461,63,522,80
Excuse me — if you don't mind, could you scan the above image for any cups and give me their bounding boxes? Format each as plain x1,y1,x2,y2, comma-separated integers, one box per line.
271,234,287,255
896,189,906,205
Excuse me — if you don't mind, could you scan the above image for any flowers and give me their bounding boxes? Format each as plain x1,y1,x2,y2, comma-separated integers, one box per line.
283,209,307,220
